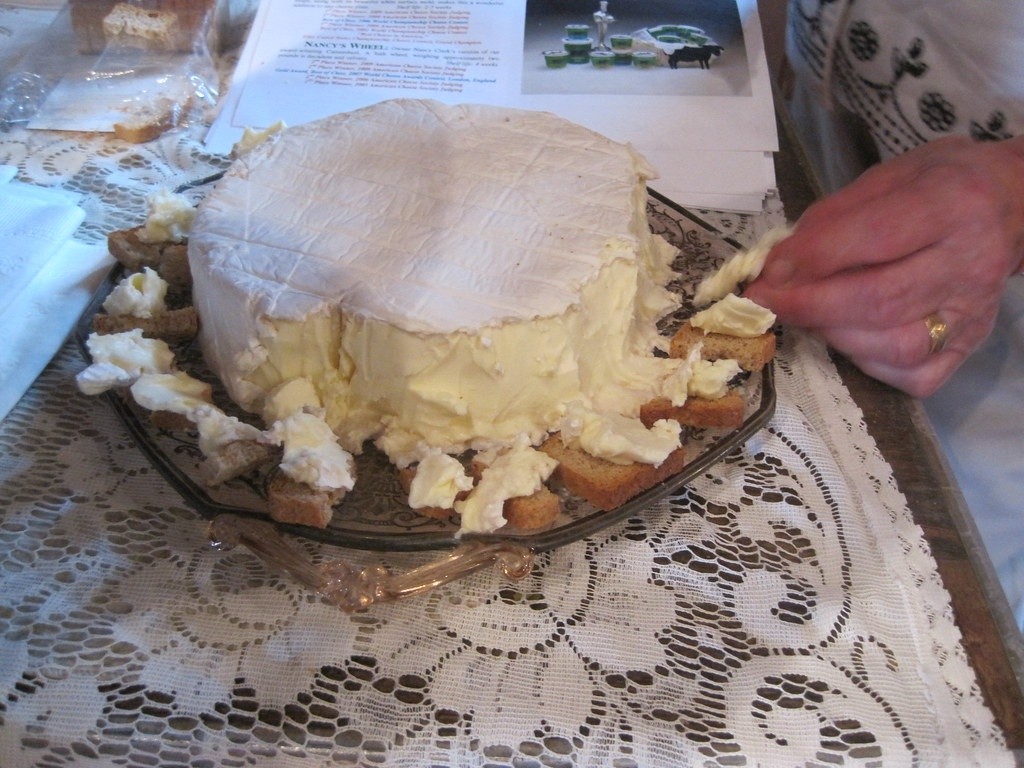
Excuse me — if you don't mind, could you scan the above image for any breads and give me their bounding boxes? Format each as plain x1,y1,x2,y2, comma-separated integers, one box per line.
102,2,179,40
112,91,194,141
67,0,221,52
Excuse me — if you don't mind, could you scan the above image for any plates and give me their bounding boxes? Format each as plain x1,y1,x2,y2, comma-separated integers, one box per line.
75,165,779,609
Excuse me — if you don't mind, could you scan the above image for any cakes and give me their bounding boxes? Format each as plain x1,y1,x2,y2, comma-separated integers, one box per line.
72,97,793,531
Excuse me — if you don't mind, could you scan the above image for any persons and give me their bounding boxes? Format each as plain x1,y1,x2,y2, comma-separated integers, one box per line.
742,0,1023,634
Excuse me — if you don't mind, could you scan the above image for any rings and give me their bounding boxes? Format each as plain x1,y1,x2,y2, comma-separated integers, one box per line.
923,313,949,355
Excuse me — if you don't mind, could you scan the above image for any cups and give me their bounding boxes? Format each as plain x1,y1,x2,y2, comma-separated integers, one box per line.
543,25,656,69
